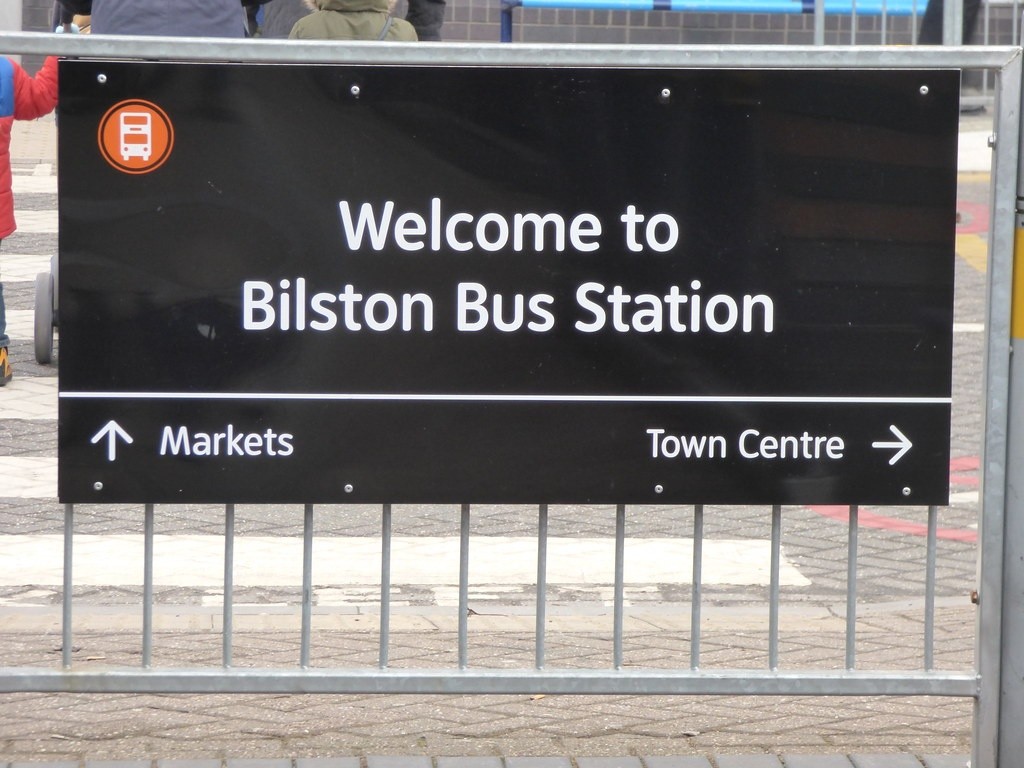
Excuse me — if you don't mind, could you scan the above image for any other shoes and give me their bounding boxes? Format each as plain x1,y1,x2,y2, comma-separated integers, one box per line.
0,346,13,386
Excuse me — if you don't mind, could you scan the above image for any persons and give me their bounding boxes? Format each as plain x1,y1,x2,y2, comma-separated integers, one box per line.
53,0,246,40
241,0,446,41
0,24,81,387
